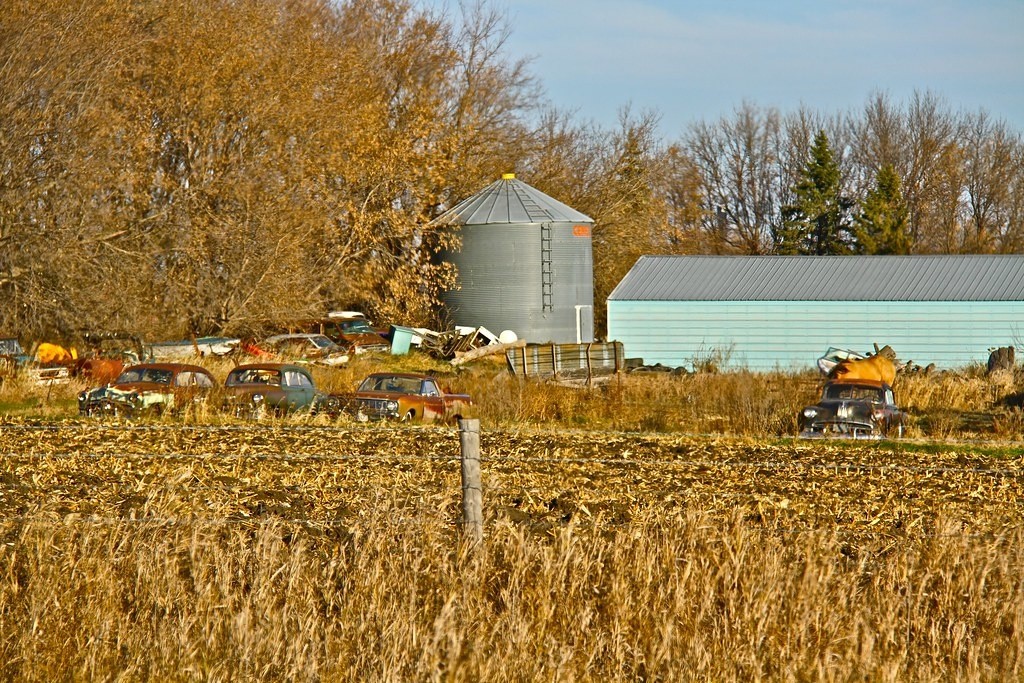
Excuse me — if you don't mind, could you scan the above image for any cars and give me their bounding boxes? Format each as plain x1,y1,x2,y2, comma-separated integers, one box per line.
219,364,325,415
802,378,906,436
267,312,391,368
77,363,223,416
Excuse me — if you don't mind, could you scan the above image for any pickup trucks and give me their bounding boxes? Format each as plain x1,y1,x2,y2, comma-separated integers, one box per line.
329,372,472,427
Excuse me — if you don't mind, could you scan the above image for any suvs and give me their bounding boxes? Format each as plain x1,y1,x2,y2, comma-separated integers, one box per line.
0,339,71,388
